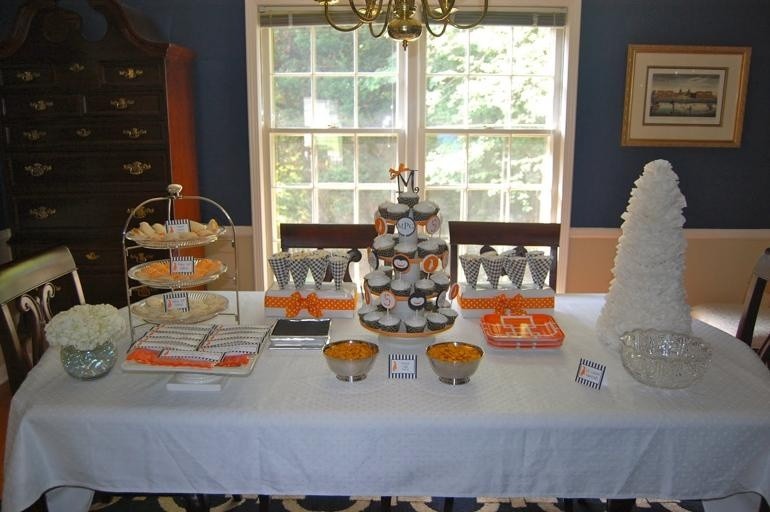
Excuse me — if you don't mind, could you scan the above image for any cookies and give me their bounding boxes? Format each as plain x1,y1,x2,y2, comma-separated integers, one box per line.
127,219,224,320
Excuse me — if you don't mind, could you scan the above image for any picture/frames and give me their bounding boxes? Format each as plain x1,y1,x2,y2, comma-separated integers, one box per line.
620,44,752,149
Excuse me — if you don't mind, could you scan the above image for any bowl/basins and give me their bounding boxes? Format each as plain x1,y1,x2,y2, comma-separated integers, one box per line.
618,327,713,390
322,340,379,382
425,341,485,385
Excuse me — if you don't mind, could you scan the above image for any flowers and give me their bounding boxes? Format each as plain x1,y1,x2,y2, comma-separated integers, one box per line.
39,302,125,352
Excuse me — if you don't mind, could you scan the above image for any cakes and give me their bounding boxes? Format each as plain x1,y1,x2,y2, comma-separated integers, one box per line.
358,192,554,332
269,248,350,291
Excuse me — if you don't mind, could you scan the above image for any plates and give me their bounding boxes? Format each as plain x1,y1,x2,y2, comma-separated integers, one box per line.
480,313,565,347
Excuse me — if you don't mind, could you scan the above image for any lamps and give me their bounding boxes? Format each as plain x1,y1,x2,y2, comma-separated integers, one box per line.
315,0,490,50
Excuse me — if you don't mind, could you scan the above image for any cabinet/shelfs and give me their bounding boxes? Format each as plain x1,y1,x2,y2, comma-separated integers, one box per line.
116,183,240,347
2,3,207,311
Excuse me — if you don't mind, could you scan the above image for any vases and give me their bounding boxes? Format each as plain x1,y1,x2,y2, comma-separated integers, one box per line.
57,343,118,381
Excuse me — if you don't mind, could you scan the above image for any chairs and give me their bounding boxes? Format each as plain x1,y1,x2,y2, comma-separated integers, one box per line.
277,223,563,295
734,248,769,369
0,242,87,396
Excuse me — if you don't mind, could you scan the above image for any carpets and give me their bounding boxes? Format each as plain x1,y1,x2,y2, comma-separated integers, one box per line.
93,489,703,511
